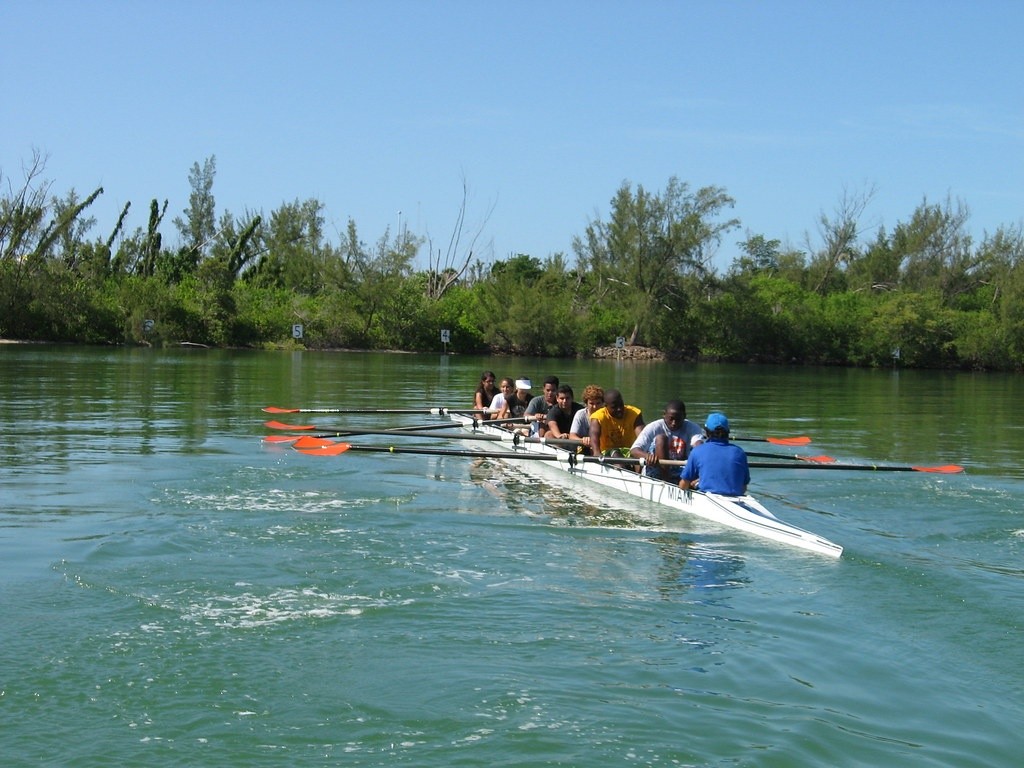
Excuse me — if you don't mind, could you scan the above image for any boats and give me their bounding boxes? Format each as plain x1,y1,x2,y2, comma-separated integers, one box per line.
448,411,844,560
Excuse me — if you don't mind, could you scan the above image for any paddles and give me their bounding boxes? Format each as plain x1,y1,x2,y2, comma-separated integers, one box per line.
260,405,964,475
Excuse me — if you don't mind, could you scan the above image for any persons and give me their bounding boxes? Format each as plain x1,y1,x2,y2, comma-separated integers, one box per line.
497,376,534,436
544,385,584,451
570,384,605,455
679,413,751,496
589,389,646,474
524,375,559,437
489,377,514,419
630,400,706,483
473,371,500,420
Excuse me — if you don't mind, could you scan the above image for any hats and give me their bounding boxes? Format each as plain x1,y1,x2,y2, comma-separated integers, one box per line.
516,380,531,389
705,413,729,431
